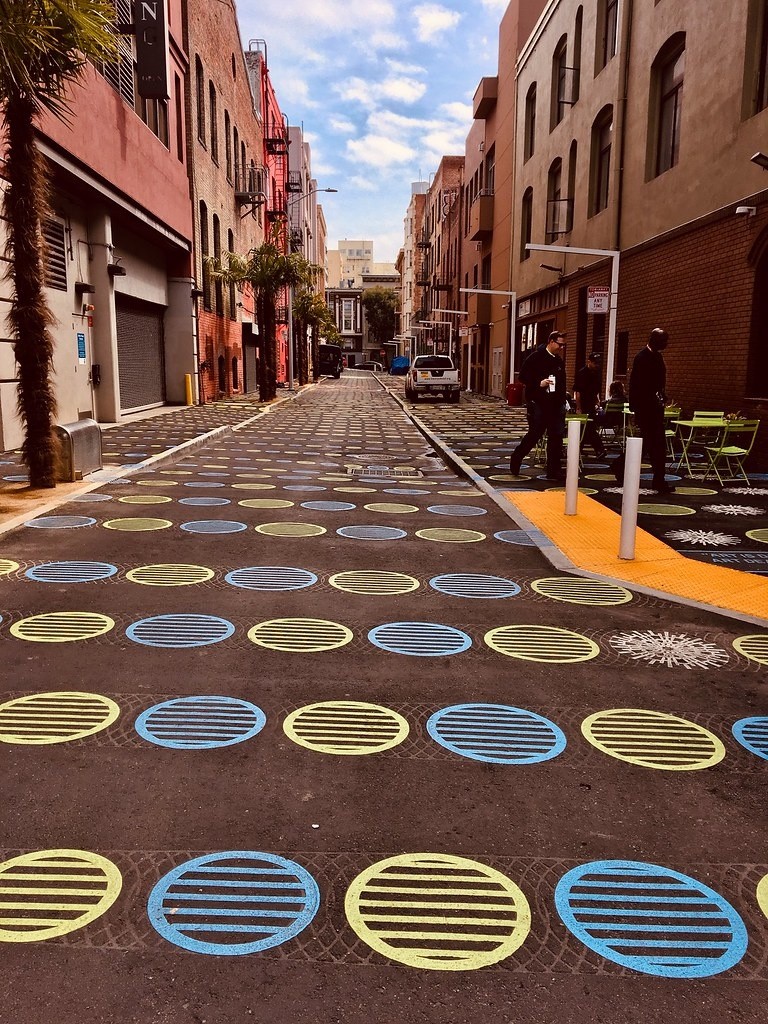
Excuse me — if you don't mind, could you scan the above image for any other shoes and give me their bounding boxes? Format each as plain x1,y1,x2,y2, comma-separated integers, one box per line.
651,479,676,492
609,461,624,482
546,472,566,481
510,453,521,476
596,451,608,460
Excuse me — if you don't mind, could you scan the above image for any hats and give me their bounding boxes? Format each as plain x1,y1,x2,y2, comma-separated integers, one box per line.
589,351,604,363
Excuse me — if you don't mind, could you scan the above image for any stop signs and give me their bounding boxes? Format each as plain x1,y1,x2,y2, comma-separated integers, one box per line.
380,349,387,358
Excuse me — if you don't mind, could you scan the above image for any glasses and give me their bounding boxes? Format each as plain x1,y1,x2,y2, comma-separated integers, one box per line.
554,340,567,348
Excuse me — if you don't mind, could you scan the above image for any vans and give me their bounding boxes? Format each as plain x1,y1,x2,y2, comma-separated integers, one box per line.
318,344,344,379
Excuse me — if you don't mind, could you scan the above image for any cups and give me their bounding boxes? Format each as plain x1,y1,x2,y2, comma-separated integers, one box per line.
548,374,556,392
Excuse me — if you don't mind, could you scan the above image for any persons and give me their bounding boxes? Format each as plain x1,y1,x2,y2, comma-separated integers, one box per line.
610,328,677,491
510,330,566,481
602,381,628,428
573,353,608,460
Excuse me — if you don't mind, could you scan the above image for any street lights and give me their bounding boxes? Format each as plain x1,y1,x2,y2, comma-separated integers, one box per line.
287,187,339,391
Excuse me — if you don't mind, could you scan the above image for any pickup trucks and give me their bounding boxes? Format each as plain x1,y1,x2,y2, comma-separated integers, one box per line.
404,355,461,403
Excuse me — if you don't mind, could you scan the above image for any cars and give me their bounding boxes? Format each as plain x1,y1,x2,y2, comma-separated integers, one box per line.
354,361,386,372
